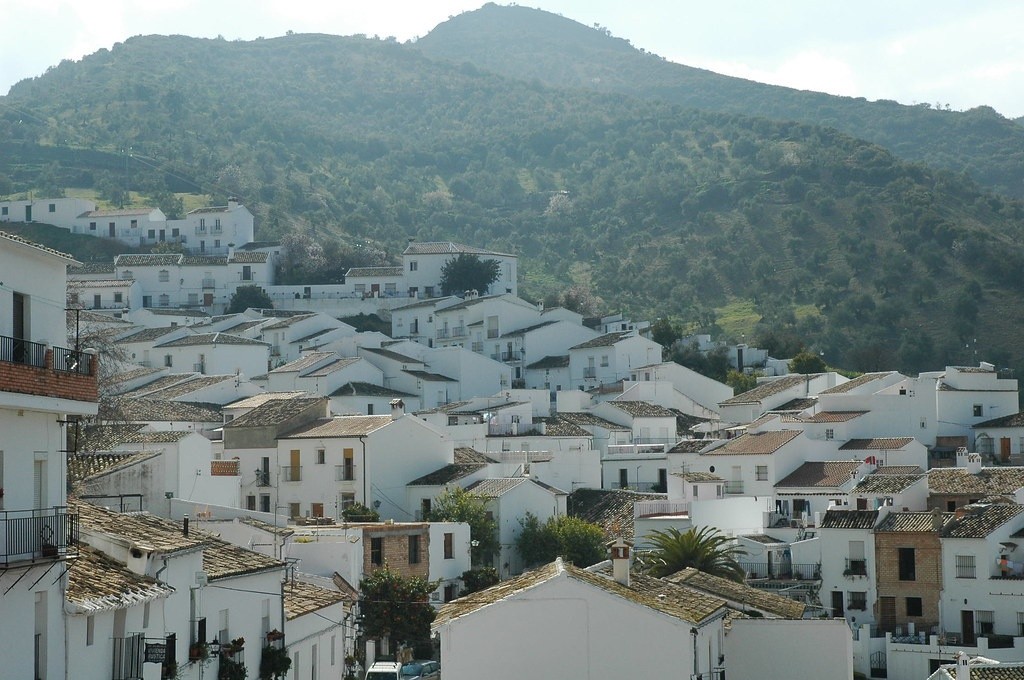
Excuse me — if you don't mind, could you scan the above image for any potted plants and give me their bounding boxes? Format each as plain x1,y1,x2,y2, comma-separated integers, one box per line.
190,641,212,664
222,642,232,652
43,543,58,557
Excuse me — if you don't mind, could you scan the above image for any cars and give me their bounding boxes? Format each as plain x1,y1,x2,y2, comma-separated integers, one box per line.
401,659,441,680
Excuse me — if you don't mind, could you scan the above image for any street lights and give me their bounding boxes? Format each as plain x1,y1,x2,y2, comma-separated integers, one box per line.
468,540,479,553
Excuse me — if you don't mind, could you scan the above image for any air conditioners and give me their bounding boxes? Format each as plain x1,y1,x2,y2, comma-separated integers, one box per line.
790,519,801,528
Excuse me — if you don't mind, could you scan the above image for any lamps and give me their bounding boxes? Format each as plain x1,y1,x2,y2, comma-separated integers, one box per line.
208,636,220,658
357,611,366,621
254,468,261,476
467,539,480,553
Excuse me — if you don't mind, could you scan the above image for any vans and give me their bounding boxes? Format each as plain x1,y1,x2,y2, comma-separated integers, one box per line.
365,661,402,680
